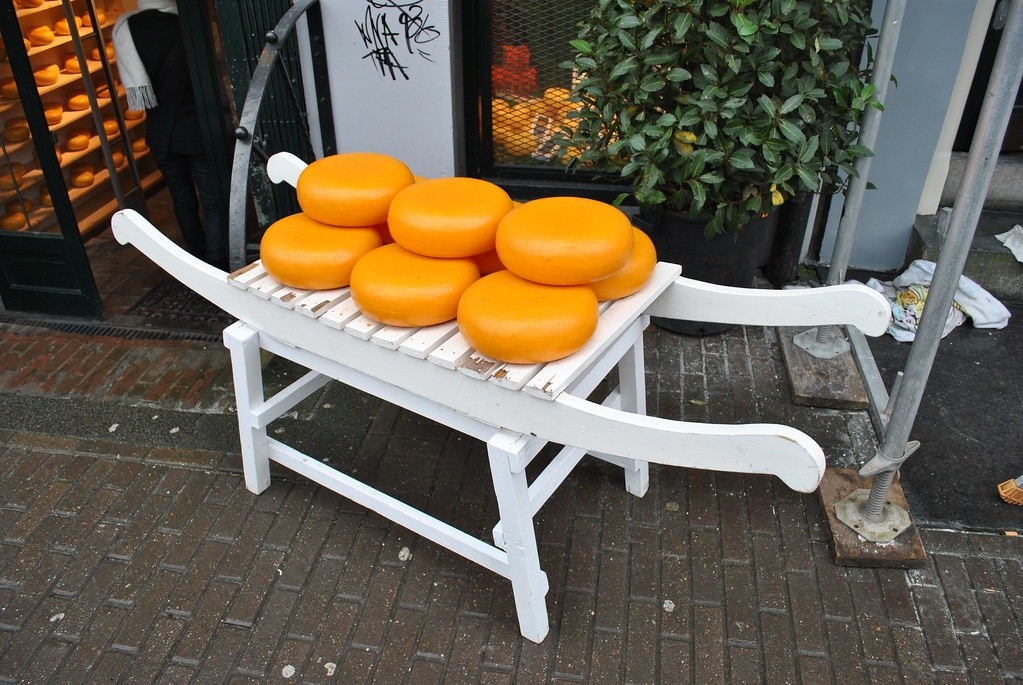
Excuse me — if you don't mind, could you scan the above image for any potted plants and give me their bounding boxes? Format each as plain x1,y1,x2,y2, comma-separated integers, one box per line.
538,0,898,341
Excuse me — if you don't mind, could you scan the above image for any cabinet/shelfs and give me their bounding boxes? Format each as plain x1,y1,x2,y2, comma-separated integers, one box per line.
0,0,165,237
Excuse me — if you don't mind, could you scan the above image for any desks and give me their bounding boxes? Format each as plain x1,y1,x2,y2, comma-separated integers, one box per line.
110,152,891,644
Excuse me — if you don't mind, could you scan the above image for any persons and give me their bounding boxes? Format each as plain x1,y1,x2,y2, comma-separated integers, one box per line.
112,0,228,265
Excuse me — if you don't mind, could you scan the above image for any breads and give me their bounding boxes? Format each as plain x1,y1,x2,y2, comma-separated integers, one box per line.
260,153,657,364
0,0,149,231
490,84,621,166
